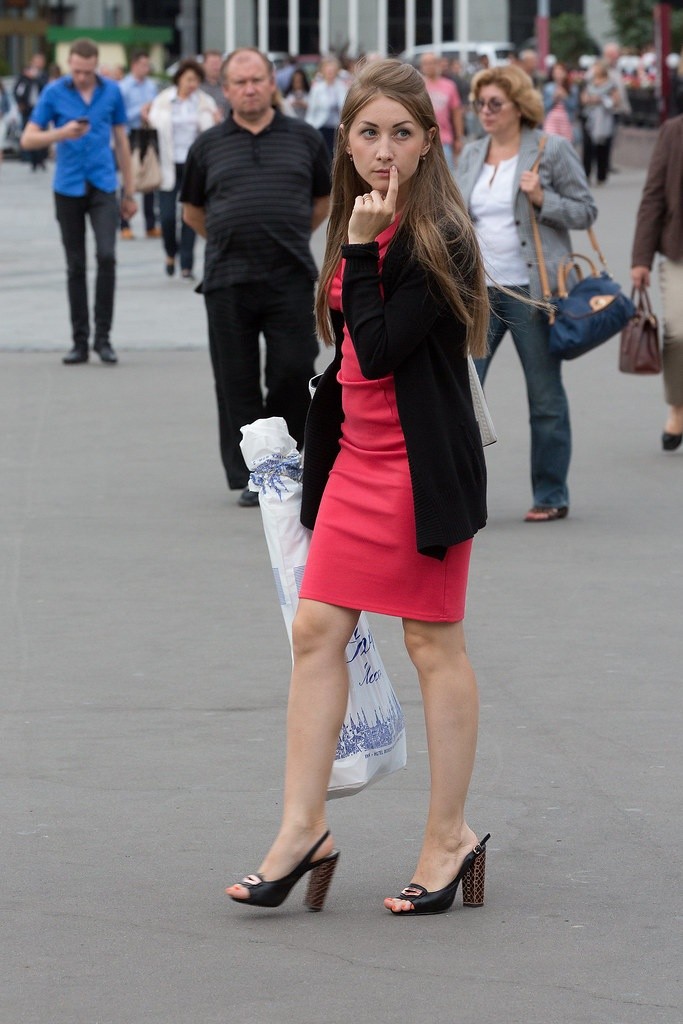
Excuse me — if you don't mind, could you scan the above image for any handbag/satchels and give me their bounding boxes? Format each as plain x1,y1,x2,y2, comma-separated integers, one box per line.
542,252,638,363
306,342,498,451
617,283,663,376
127,118,163,195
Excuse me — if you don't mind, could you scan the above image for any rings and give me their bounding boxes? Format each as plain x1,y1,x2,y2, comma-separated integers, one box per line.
363,194,373,201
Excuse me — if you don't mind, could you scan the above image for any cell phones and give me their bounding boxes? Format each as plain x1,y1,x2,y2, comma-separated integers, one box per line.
77,117,91,127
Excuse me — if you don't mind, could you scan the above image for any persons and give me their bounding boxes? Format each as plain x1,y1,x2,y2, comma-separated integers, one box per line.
15,52,58,169
181,48,330,507
226,61,493,917
99,45,683,279
18,36,140,365
448,65,600,521
631,110,683,451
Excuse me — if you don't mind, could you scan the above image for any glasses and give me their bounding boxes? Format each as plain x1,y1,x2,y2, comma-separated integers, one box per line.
472,98,511,114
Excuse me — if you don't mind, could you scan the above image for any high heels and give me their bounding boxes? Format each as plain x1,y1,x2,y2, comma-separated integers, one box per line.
227,828,342,913
387,832,493,918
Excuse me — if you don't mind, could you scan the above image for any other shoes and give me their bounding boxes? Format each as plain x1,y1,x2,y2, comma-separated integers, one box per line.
121,227,134,240
238,488,259,507
93,341,117,363
661,430,683,450
166,263,174,275
182,273,191,279
62,346,89,364
147,228,161,238
595,179,604,188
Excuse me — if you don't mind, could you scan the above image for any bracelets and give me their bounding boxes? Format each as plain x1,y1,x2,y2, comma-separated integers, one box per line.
121,192,133,200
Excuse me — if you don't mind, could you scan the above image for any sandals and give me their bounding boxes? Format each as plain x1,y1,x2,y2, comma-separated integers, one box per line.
523,504,570,522
